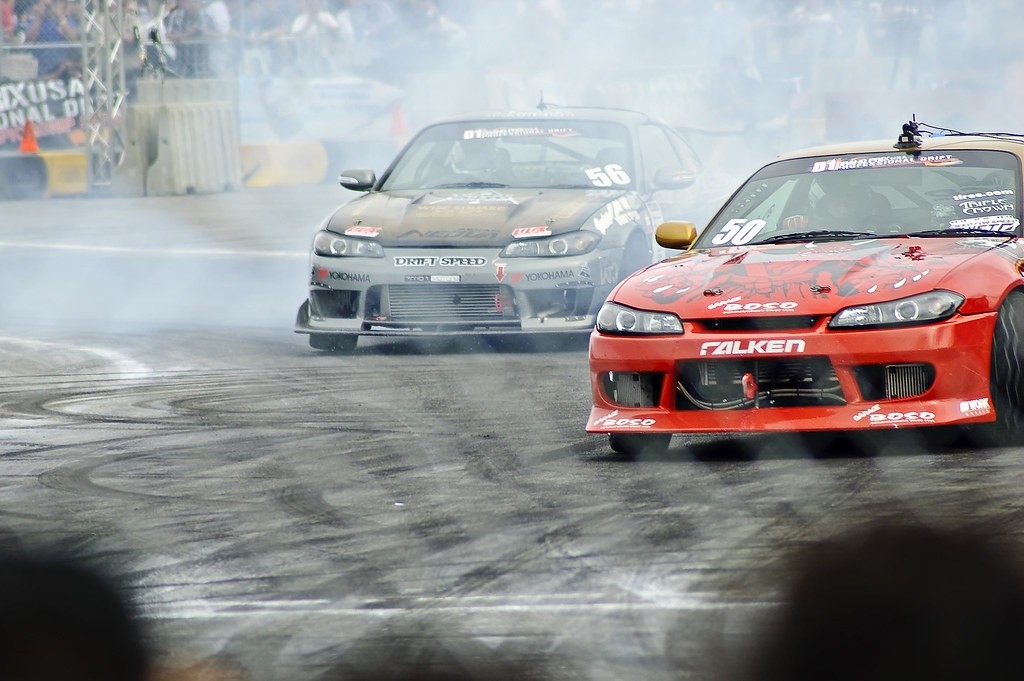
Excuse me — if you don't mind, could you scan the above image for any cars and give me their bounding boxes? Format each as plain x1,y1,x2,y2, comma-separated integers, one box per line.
583,133,1024,452
291,103,706,350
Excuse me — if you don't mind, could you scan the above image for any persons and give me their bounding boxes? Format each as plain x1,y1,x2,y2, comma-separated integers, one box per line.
432,133,513,187
813,171,892,234
0,0,933,82
